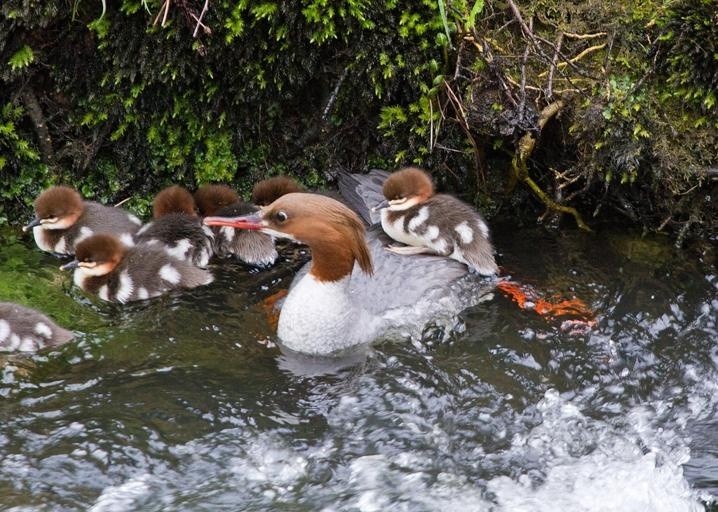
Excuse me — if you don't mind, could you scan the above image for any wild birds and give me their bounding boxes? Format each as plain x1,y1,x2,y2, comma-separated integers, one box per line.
193,182,279,270
203,156,594,356
135,183,216,269
245,173,359,218
57,230,216,306
0,300,75,353
22,183,149,257
369,167,499,280
